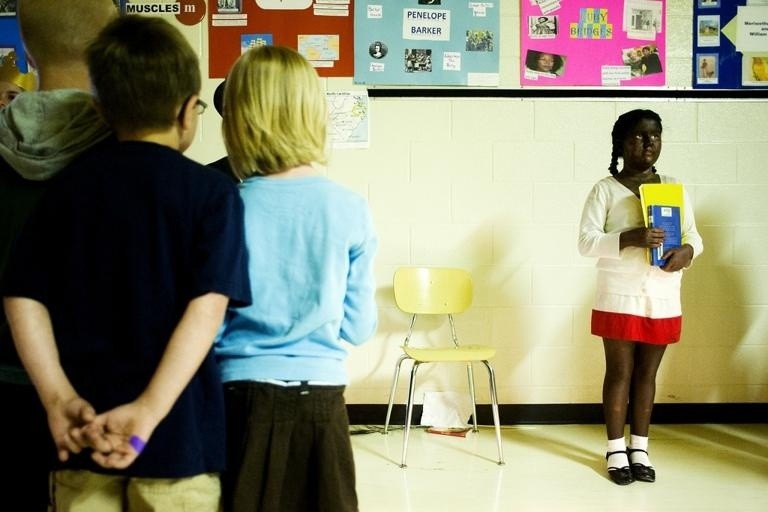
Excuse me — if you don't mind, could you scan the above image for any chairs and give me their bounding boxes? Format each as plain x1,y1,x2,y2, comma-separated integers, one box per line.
626,446,655,482
606,451,633,486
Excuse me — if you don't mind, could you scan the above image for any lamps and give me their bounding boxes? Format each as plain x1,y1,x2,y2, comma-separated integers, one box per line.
383,266,505,467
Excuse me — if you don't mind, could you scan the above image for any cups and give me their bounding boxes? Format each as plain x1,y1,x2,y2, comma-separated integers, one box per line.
197,98,207,114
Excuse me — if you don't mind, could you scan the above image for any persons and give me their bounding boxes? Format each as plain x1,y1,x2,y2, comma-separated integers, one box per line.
0,49,40,115
0,0,121,512
204,44,380,512
0,13,254,512
701,58,709,78
578,108,704,486
535,52,555,74
624,46,661,74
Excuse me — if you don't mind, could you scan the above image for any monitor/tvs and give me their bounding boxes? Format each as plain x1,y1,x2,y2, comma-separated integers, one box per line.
637,182,685,267
426,427,473,437
